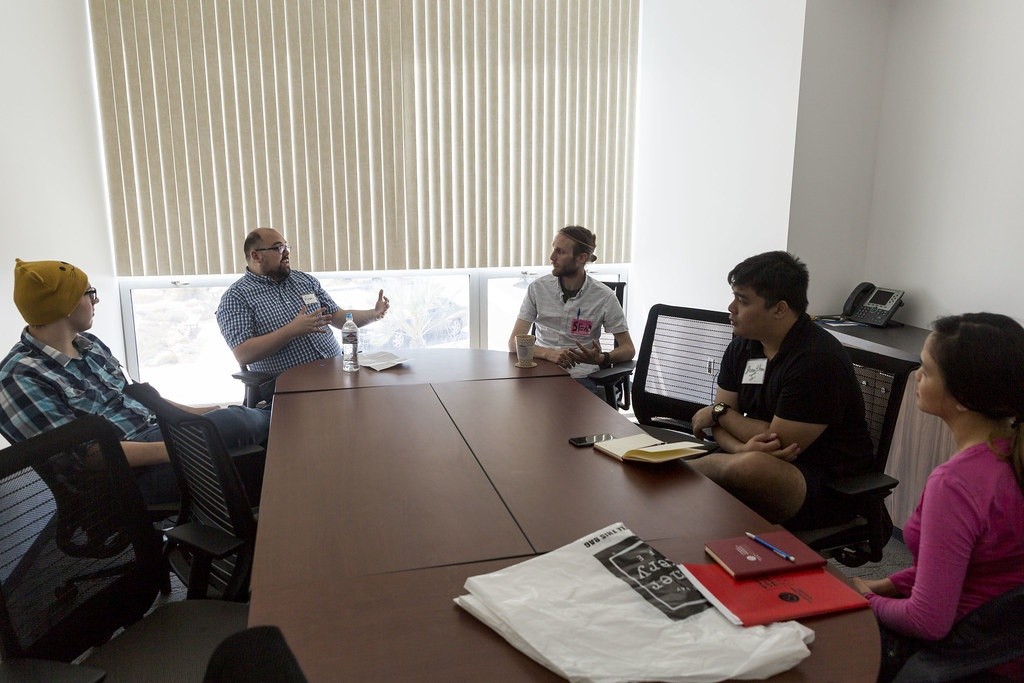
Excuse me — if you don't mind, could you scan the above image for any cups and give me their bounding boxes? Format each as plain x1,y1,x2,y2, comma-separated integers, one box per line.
515,335,535,366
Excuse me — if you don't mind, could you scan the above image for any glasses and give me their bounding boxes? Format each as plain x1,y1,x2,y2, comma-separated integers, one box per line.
83,287,97,300
253,244,292,254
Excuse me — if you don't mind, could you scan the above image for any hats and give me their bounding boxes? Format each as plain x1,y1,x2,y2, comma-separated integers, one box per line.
14,258,87,326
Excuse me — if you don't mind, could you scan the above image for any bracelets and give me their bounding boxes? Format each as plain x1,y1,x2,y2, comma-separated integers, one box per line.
598,352,610,368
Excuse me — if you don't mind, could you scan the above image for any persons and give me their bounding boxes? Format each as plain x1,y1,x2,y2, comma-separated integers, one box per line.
508,225,635,396
853,314,1024,683
0,257,272,507
215,228,389,376
683,251,876,531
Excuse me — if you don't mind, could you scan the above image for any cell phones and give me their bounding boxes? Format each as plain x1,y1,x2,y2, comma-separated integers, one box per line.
569,433,618,446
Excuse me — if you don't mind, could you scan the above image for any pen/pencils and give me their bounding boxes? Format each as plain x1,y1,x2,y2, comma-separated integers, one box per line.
744,531,796,562
636,442,665,448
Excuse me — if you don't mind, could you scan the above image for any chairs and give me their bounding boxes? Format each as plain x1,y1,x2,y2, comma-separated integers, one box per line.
587,281,636,411
630,303,737,454
0,370,276,683
881,588,1024,683
784,351,922,570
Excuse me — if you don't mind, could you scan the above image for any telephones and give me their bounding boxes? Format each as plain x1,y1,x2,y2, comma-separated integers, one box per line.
840,282,905,327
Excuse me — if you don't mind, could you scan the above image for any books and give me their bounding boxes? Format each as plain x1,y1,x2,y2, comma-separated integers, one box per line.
705,529,827,580
677,563,870,628
594,434,708,465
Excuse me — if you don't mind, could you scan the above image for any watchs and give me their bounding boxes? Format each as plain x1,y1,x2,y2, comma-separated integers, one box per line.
711,402,730,425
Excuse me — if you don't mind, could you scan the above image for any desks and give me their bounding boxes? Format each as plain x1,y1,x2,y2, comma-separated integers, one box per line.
245,345,880,683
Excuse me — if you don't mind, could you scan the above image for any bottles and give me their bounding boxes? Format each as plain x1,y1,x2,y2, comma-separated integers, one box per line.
342,314,359,373
